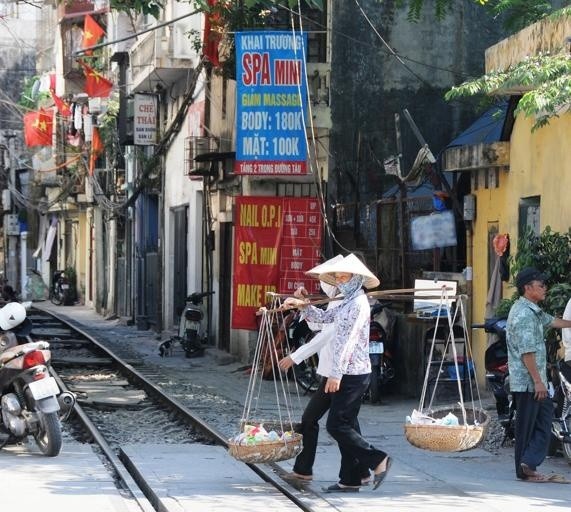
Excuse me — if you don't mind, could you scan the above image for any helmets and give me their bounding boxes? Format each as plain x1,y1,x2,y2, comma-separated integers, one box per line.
0,302,27,331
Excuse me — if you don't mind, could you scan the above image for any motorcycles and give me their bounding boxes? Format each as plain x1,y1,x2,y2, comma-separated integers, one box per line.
467,310,571,466
178,288,216,356
0,299,77,464
49,269,73,306
356,301,398,405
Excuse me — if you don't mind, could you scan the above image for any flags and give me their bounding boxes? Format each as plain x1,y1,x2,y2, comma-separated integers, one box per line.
23,12,115,162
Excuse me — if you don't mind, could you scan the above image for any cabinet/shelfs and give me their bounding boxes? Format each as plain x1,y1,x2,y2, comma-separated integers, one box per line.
423,325,467,406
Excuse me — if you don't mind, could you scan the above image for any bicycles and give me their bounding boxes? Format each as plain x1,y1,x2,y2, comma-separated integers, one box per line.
281,295,326,394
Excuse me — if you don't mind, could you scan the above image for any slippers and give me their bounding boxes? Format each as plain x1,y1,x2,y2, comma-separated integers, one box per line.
515,473,544,482
545,473,570,483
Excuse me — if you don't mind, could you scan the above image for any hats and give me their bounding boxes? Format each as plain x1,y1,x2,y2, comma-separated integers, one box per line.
305,254,344,279
516,267,550,287
319,253,381,290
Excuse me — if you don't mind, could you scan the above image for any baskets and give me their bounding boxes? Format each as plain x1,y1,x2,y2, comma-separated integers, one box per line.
405,405,490,452
228,419,305,462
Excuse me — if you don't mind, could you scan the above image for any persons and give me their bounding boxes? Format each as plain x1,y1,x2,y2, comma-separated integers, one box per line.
560,298,571,384
282,252,395,495
278,254,373,487
505,267,571,482
